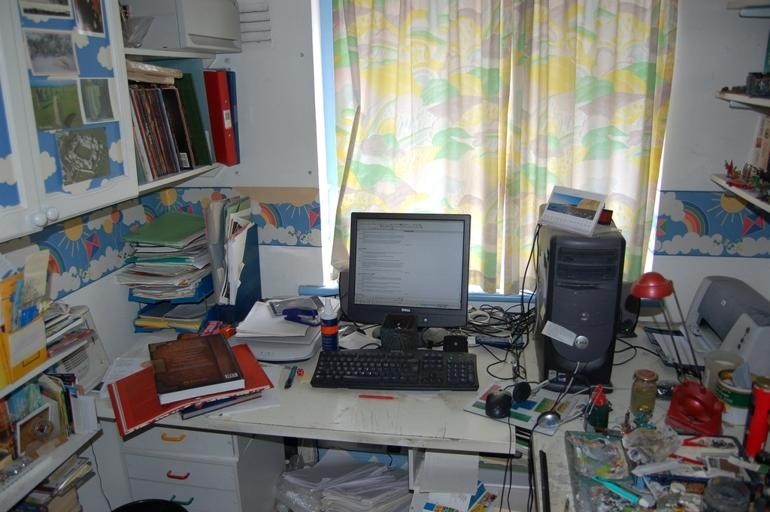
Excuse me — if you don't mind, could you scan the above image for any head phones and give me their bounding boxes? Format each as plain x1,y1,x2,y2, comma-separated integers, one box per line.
513,373,592,429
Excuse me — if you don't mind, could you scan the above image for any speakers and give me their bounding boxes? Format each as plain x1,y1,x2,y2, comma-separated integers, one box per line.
338,269,354,322
618,281,641,338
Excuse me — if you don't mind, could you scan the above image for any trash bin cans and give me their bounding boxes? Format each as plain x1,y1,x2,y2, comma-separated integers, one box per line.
111,499,189,512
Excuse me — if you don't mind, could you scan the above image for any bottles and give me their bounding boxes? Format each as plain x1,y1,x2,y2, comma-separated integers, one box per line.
744,375,769,461
319,299,339,350
586,385,610,435
630,367,659,419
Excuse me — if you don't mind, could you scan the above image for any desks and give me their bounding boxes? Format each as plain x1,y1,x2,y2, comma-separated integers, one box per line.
90,299,770,512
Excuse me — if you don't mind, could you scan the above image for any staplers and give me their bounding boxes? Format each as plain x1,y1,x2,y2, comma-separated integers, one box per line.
283,307,321,326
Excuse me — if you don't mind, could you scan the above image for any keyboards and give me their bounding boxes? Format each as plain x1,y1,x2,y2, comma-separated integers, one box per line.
310,349,479,392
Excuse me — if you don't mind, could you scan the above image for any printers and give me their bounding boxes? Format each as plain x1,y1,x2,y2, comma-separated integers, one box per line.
645,274,770,379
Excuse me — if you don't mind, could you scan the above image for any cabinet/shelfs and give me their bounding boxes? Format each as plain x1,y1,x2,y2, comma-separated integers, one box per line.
124,271,222,337
1,0,140,249
703,1,770,217
0,328,106,512
116,48,222,197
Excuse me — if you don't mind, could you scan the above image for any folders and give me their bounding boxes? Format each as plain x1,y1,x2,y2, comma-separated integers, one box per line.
203,68,238,167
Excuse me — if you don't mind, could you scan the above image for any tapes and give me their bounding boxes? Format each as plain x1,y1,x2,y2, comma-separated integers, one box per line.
468,309,491,325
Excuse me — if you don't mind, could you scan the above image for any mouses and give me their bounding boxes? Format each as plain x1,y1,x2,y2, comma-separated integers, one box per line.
486,389,511,419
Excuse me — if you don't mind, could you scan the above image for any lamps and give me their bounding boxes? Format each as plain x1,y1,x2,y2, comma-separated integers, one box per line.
627,269,726,438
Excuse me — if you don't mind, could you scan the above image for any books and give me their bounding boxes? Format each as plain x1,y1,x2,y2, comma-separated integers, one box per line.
182,392,262,420
126,58,260,330
536,184,608,238
147,332,246,407
1,310,95,512
107,344,274,437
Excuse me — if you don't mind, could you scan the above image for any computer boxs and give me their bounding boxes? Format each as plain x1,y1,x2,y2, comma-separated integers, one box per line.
538,204,626,395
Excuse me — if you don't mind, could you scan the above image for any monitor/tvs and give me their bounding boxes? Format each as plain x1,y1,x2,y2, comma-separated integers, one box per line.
347,212,471,350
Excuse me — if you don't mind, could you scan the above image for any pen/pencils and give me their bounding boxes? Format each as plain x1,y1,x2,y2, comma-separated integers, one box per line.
591,476,650,508
285,366,297,389
358,394,396,400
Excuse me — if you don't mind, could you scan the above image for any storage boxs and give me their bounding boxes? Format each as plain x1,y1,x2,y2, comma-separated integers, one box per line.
1,312,49,388
